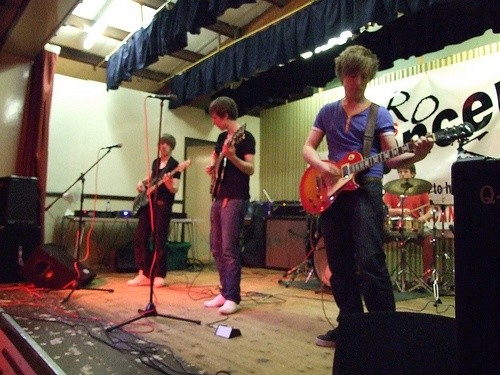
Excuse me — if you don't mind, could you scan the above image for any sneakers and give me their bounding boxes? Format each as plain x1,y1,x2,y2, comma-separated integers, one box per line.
315,328,338,348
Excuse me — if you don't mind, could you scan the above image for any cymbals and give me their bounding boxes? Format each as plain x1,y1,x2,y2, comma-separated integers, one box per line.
383,177,434,196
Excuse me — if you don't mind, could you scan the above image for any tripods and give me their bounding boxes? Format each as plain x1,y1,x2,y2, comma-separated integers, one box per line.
106,99,201,332
391,186,454,306
45,148,114,303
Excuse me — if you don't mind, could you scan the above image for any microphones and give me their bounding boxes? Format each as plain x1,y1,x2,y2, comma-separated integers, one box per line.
106,144,122,148
148,94,178,100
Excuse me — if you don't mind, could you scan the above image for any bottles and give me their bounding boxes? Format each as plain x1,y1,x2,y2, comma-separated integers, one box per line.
106,200,111,211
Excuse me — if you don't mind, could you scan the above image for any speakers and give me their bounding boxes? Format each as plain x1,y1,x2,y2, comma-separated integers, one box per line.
263,216,314,269
24,243,96,292
0,176,42,281
331,158,500,375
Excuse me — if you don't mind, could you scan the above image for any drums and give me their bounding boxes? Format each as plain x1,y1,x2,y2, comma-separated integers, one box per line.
311,235,333,288
384,216,426,240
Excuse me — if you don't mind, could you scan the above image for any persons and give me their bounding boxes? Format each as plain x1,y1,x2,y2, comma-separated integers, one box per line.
126,134,181,287
203,96,256,314
301,45,434,347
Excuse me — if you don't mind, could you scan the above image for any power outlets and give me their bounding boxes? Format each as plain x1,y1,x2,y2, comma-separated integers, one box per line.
215,324,242,339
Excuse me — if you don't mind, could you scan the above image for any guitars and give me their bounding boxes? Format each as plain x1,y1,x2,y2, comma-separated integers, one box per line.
299,121,475,216
132,156,194,216
209,121,250,202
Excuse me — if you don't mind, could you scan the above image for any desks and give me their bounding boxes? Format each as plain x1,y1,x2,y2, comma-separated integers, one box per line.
66,216,192,263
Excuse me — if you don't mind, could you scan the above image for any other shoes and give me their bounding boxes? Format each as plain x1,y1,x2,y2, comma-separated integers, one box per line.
153,277,165,288
203,294,240,314
127,275,151,286
